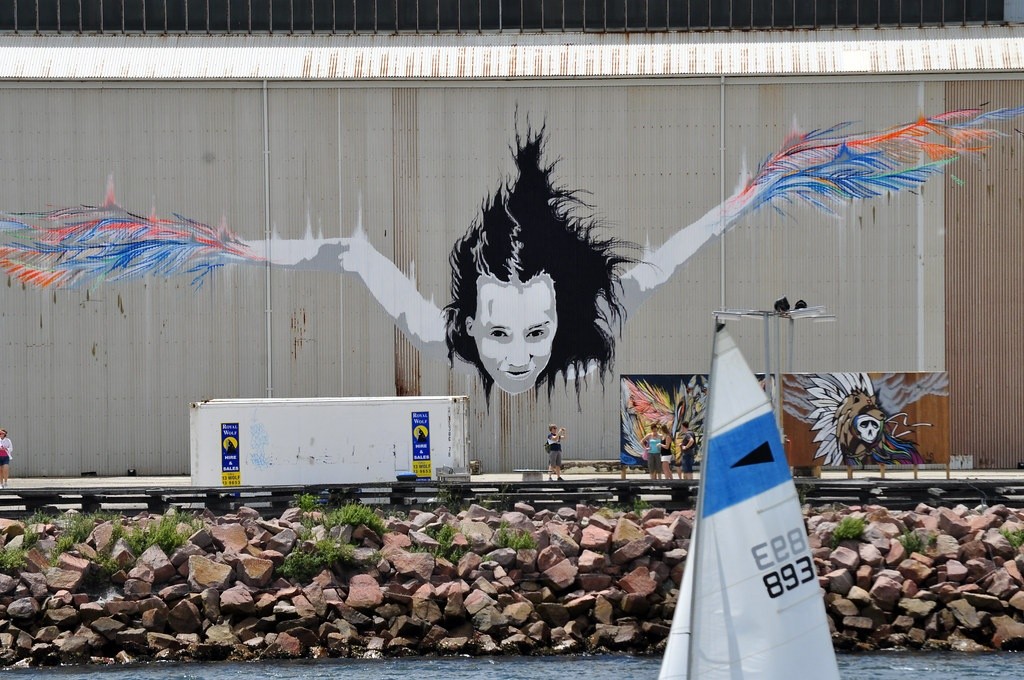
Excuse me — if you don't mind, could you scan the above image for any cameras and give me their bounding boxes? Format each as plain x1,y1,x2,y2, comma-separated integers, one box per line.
562,428,565,431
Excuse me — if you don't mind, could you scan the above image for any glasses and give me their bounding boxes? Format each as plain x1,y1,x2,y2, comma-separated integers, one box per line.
554,427,557,429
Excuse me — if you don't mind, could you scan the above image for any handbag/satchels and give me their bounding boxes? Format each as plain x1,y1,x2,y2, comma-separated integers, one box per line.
544,441,550,453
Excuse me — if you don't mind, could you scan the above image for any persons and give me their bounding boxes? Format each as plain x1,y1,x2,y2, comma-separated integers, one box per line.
640,424,664,479
680,421,695,479
547,424,566,482
0,430,13,488
657,425,673,479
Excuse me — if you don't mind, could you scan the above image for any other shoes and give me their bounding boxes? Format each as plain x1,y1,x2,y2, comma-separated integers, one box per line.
557,477,565,481
548,478,553,481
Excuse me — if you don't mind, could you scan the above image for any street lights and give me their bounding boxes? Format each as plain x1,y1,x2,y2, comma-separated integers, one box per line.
715,296,837,478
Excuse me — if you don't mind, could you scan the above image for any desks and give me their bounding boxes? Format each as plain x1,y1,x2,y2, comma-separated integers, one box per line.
513,469,553,482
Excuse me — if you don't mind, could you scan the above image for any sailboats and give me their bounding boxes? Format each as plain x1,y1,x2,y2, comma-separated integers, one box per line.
656,316,843,679
